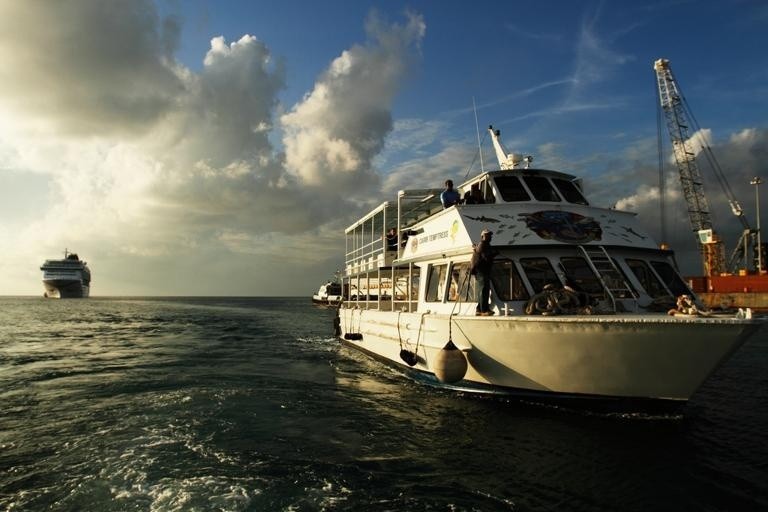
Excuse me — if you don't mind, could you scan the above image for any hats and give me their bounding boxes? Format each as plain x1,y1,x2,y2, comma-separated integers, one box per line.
481,229,493,236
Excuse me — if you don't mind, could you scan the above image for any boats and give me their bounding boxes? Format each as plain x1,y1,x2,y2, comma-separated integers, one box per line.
643,55,768,319
332,89,764,429
310,267,344,304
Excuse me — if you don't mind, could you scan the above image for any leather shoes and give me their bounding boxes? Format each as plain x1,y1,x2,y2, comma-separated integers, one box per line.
476,306,494,316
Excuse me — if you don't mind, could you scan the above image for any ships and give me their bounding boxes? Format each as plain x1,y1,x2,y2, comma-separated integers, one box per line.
39,245,93,299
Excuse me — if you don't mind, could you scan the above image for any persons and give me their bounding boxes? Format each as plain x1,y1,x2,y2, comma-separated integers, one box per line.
386,229,397,251
471,229,500,316
440,180,484,210
